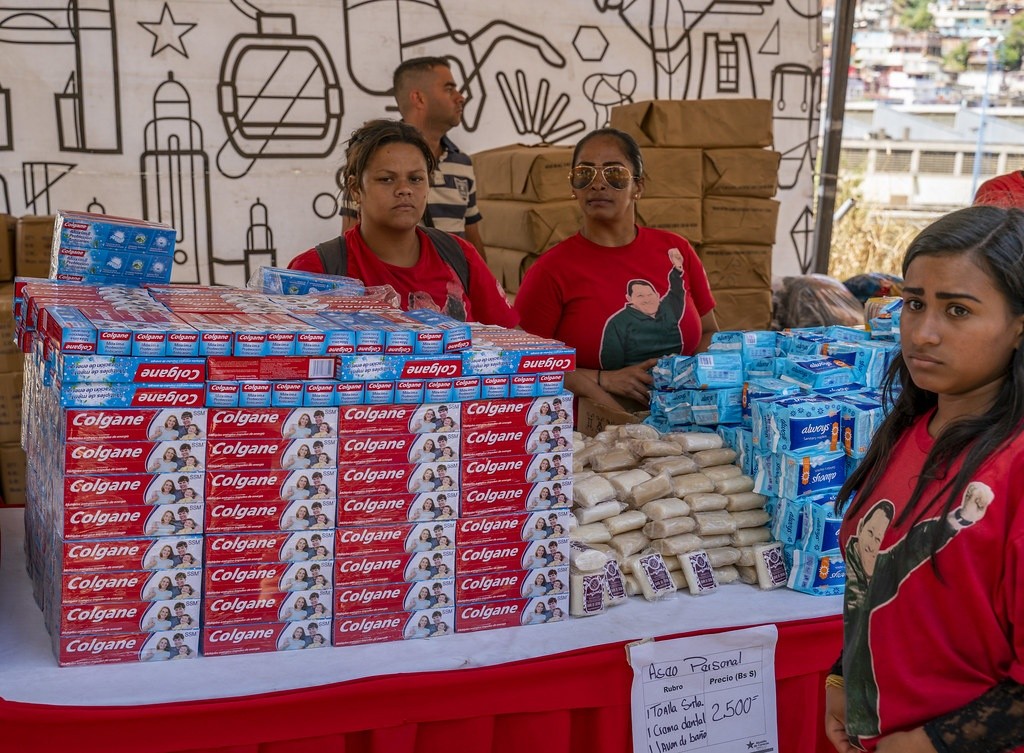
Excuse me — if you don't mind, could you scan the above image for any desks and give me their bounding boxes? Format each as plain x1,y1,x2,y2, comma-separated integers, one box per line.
0,506,846,752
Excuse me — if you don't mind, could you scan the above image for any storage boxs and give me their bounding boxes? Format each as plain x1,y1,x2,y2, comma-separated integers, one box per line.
0,94,781,669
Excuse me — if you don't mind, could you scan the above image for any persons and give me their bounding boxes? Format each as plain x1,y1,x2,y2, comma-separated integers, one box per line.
404,406,459,637
973,170,1024,210
825,207,1024,753
524,398,572,623
515,129,719,435
281,410,337,648
287,119,623,411
339,56,487,265
141,412,205,661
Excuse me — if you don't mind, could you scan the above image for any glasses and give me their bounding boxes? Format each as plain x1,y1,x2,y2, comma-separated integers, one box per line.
566,165,639,192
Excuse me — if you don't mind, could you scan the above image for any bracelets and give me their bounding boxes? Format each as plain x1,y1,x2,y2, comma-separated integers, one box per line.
597,369,601,386
826,676,844,689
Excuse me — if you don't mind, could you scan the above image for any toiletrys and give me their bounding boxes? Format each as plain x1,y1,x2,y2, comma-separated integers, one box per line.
5,204,578,668
